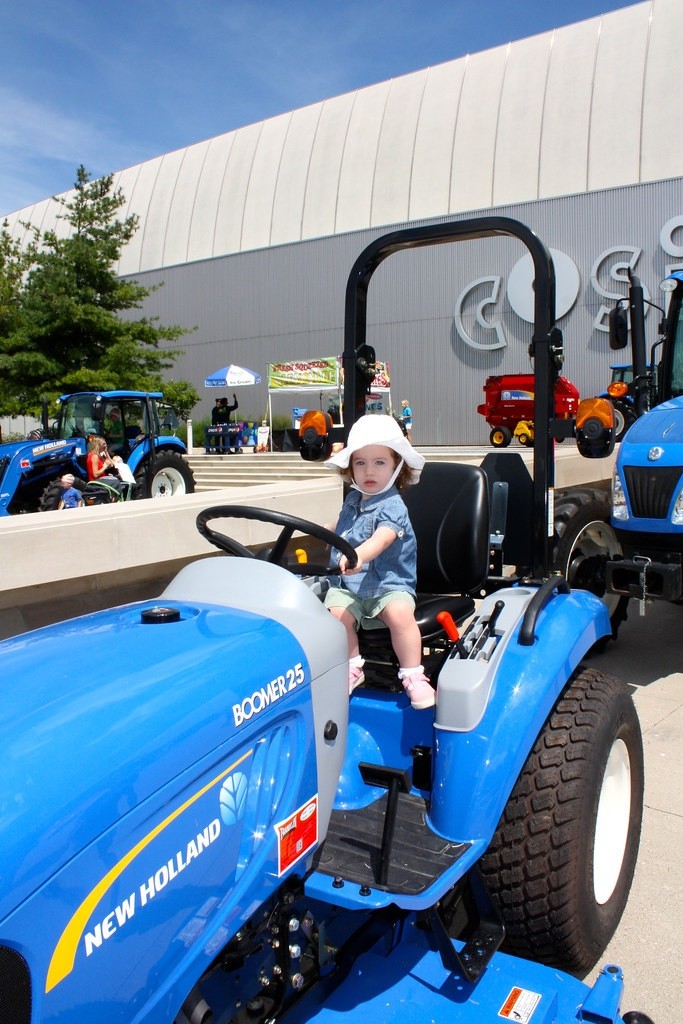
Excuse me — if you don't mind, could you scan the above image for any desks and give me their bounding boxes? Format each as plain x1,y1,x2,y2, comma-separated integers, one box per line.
273,428,346,453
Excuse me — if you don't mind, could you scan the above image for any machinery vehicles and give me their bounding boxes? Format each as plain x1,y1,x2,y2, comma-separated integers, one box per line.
0,391,197,519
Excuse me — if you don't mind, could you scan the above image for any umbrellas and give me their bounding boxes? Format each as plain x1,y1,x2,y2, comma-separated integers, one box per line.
204,363,262,423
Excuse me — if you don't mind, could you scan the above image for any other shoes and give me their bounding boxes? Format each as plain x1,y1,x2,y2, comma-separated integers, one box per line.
225,450,231,453
215,449,221,454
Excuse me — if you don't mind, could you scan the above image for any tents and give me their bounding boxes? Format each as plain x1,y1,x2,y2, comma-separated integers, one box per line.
266,354,390,452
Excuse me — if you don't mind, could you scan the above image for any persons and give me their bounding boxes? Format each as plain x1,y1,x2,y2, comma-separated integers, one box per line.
211,393,238,454
386,399,413,444
323,415,437,710
86,437,122,503
58,473,82,510
327,399,340,424
105,408,125,456
66,402,96,437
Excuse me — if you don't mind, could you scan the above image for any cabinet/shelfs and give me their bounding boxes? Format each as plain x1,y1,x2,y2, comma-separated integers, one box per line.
203,424,241,455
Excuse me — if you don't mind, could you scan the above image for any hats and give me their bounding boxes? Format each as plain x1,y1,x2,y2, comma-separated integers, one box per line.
110,408,121,418
322,414,425,485
61,474,75,485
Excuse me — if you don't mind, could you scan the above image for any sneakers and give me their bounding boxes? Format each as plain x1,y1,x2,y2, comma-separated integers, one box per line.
348,658,366,695
403,674,437,710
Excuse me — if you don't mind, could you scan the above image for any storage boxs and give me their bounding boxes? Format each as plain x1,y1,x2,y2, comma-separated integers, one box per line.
332,442,345,454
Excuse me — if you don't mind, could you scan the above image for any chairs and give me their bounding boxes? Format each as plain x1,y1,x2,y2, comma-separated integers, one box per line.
359,462,491,674
113,424,142,460
